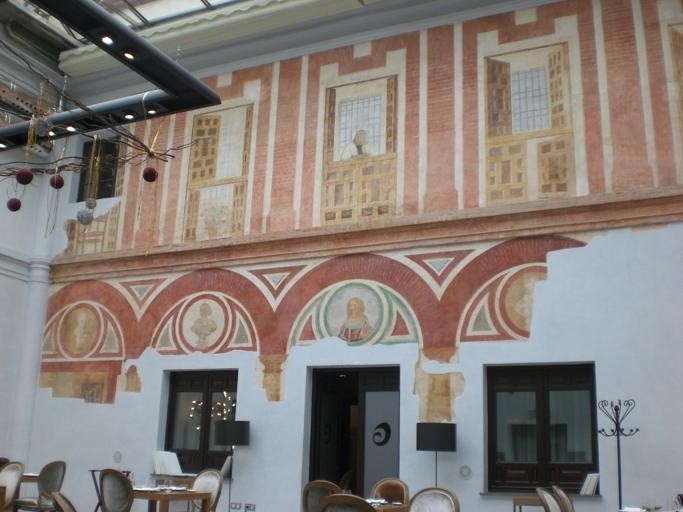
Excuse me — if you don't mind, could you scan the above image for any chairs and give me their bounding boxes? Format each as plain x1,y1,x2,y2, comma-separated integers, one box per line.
536,485,575,512
303,477,460,512
0,456,223,512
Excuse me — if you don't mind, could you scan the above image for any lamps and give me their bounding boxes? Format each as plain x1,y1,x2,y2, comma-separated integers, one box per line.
416,423,456,488
214,420,249,512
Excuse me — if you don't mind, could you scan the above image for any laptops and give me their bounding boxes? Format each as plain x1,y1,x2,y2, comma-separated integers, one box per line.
153,450,197,476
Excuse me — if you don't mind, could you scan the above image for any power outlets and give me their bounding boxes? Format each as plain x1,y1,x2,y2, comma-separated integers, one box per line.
245,504,255,510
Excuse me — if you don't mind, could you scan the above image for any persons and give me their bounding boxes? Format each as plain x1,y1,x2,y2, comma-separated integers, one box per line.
336,296,374,341
190,303,216,335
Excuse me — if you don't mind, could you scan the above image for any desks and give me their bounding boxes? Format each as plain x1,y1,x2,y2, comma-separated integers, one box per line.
513,496,545,512
